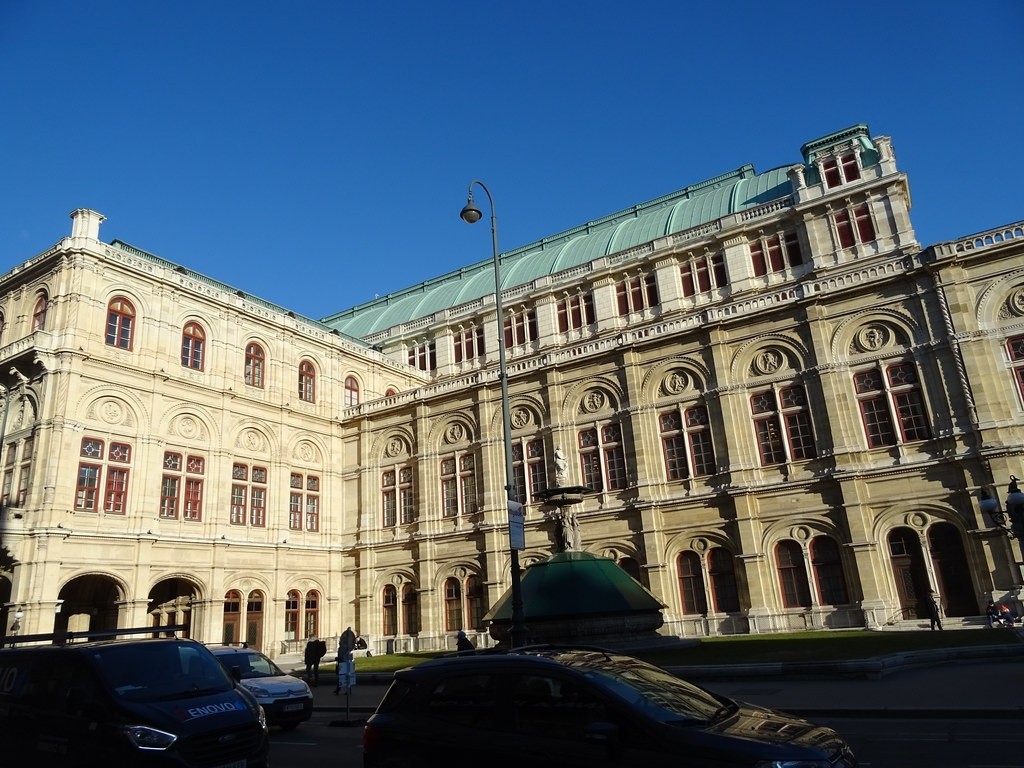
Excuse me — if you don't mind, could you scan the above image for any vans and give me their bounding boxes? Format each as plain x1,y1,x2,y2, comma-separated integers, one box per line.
1,625,273,768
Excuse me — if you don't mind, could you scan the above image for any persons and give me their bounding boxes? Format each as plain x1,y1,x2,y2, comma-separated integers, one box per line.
926,588,943,631
355,636,367,649
9,620,20,648
455,630,475,651
305,639,327,687
333,647,352,695
985,600,1015,629
553,445,568,477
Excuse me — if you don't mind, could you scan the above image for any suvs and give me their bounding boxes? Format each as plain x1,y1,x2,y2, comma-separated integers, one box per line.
200,642,315,732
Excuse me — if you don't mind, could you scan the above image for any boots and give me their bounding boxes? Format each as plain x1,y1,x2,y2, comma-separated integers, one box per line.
345,686,352,695
333,687,341,695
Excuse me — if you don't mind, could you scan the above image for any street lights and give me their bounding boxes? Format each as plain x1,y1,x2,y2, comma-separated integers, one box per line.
980,475,1024,563
459,180,523,648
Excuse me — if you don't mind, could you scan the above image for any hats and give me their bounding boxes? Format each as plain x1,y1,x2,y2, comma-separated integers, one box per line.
454,631,465,638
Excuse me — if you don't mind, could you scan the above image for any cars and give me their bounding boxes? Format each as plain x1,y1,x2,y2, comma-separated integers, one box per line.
358,642,859,768
